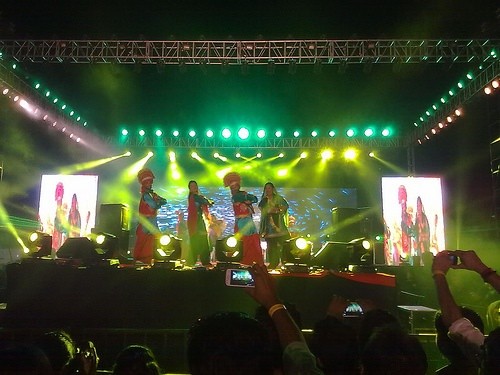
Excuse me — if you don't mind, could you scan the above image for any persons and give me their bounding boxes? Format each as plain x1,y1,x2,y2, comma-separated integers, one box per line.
176,208,225,263
257,182,299,269
384,185,442,265
187,180,215,266
0,250,500,375
45,182,93,252
223,171,265,265
133,167,167,263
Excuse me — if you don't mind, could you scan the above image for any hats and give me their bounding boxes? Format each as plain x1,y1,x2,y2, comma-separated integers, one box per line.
398,185,407,204
55,182,63,200
224,172,241,188
137,168,155,183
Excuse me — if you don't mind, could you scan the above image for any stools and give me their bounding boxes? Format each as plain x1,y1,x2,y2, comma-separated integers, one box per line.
397,305,437,337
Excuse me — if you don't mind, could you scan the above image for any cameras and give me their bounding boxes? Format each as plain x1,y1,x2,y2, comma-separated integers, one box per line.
448,255,457,266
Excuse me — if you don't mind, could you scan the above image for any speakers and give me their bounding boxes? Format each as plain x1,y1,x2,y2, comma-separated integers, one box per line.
56,238,98,259
314,241,347,267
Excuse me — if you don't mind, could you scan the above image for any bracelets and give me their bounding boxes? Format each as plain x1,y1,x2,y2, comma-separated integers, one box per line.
481,267,496,282
268,304,286,316
432,270,446,278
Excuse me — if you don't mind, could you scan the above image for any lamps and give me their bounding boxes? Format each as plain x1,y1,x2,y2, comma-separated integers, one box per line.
331,238,373,268
155,234,182,268
96,231,119,261
28,230,52,257
214,235,244,268
285,237,311,272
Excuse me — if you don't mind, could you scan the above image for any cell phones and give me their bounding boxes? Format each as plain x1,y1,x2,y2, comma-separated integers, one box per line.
225,269,255,288
343,302,363,318
